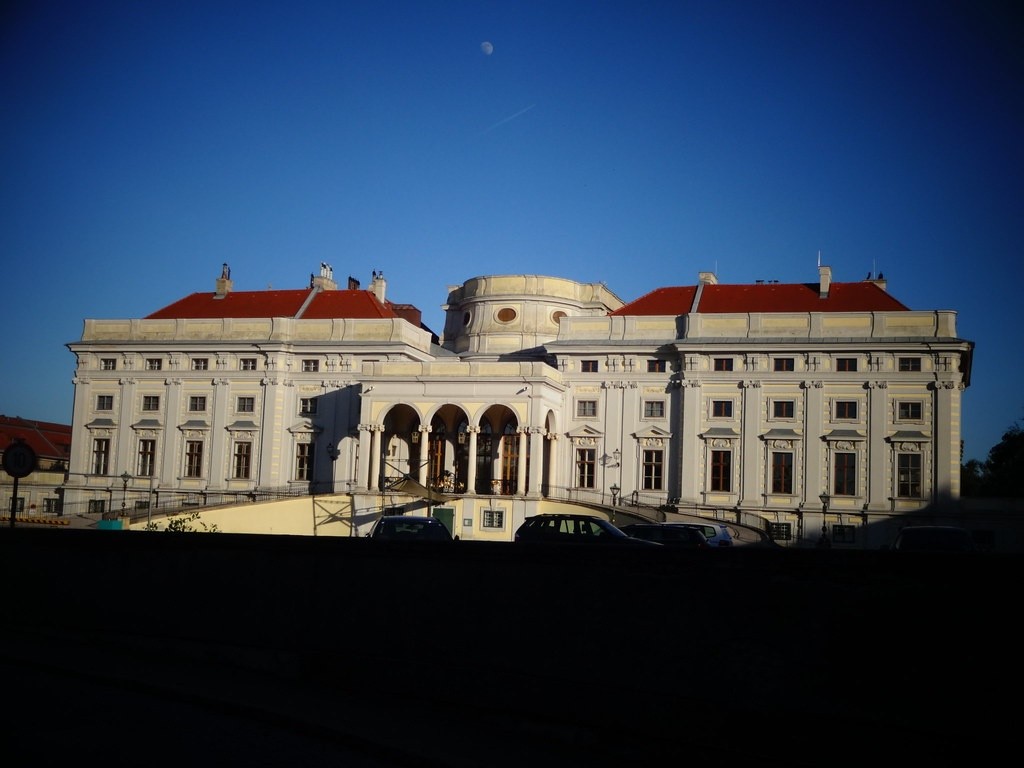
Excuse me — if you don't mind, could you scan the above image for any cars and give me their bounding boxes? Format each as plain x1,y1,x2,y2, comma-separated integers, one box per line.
611,521,752,547
365,515,459,539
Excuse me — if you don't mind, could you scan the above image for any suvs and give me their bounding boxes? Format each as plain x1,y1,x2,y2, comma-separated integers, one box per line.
516,512,664,546
